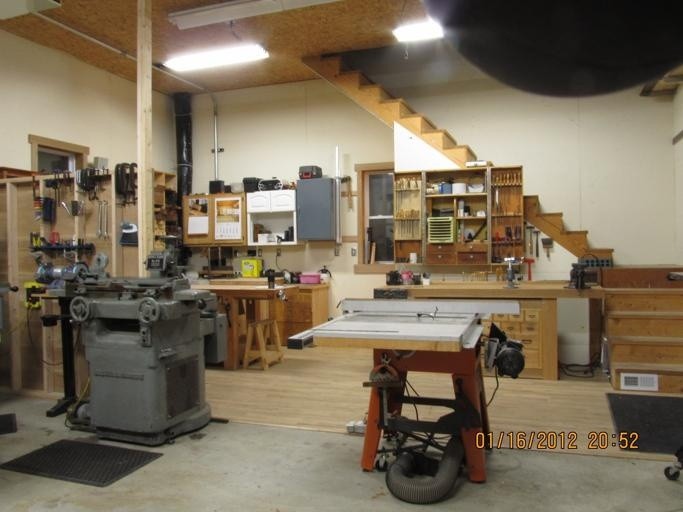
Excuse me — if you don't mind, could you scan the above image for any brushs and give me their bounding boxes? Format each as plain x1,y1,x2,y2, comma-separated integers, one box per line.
541,238,553,258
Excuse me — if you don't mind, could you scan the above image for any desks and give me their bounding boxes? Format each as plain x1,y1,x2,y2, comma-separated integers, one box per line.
191,284,297,371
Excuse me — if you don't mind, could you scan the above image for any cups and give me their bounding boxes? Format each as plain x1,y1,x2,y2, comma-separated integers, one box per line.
423,278,430,285
409,252,418,265
412,273,421,285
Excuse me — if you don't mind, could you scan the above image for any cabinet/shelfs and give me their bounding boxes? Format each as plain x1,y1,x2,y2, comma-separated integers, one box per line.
393,165,524,264
182,193,245,247
300,288,329,330
246,190,297,246
143,169,177,251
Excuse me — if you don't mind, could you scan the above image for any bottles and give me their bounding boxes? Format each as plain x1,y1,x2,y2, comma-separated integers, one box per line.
267,269,275,289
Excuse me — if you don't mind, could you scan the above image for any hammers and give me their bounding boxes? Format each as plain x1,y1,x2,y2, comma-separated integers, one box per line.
533,230,540,258
526,226,534,255
524,258,535,282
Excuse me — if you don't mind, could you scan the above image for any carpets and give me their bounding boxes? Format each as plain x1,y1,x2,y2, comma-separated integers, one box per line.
604,393,682,455
0,439,164,487
